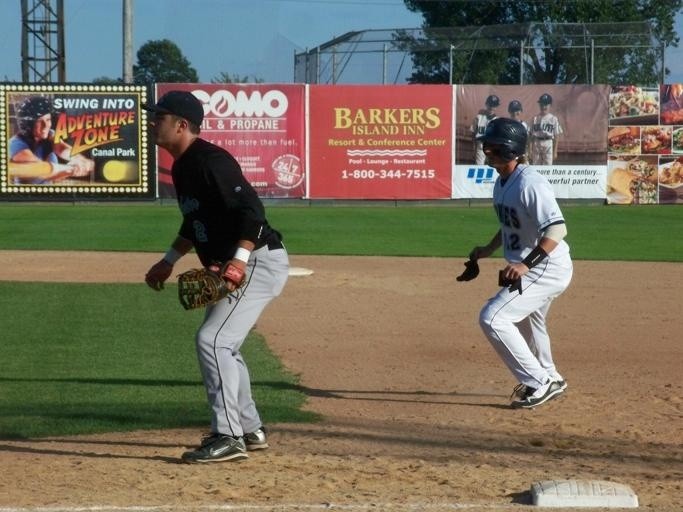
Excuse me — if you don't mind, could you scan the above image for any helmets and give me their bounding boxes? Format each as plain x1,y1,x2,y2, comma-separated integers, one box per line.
477,118,527,162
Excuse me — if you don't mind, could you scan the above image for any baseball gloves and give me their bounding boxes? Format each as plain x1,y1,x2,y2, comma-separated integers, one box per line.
177,261,246,309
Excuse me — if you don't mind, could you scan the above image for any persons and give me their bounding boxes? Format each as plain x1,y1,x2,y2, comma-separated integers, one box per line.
470,118,573,409
508,100,531,164
528,93,564,165
140,91,289,465
9,97,95,185
470,95,501,165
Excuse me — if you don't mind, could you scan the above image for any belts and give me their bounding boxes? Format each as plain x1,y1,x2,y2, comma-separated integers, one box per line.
536,136,549,140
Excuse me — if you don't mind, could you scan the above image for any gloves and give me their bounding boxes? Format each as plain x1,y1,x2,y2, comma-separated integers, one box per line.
456,261,480,281
499,269,522,294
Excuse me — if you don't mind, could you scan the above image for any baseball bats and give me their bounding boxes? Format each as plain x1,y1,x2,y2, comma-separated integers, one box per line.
10,162,73,178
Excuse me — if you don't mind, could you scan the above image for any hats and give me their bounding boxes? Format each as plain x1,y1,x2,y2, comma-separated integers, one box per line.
486,95,500,106
140,90,204,125
508,100,522,111
537,94,552,105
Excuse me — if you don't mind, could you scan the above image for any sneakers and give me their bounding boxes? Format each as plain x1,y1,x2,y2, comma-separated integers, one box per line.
510,372,568,409
181,426,270,464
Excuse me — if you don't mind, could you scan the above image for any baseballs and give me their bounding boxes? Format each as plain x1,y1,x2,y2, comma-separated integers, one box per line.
102,160,125,182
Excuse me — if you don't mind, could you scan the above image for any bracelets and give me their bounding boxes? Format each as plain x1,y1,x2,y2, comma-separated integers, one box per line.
164,247,183,265
233,247,251,264
521,244,548,269
